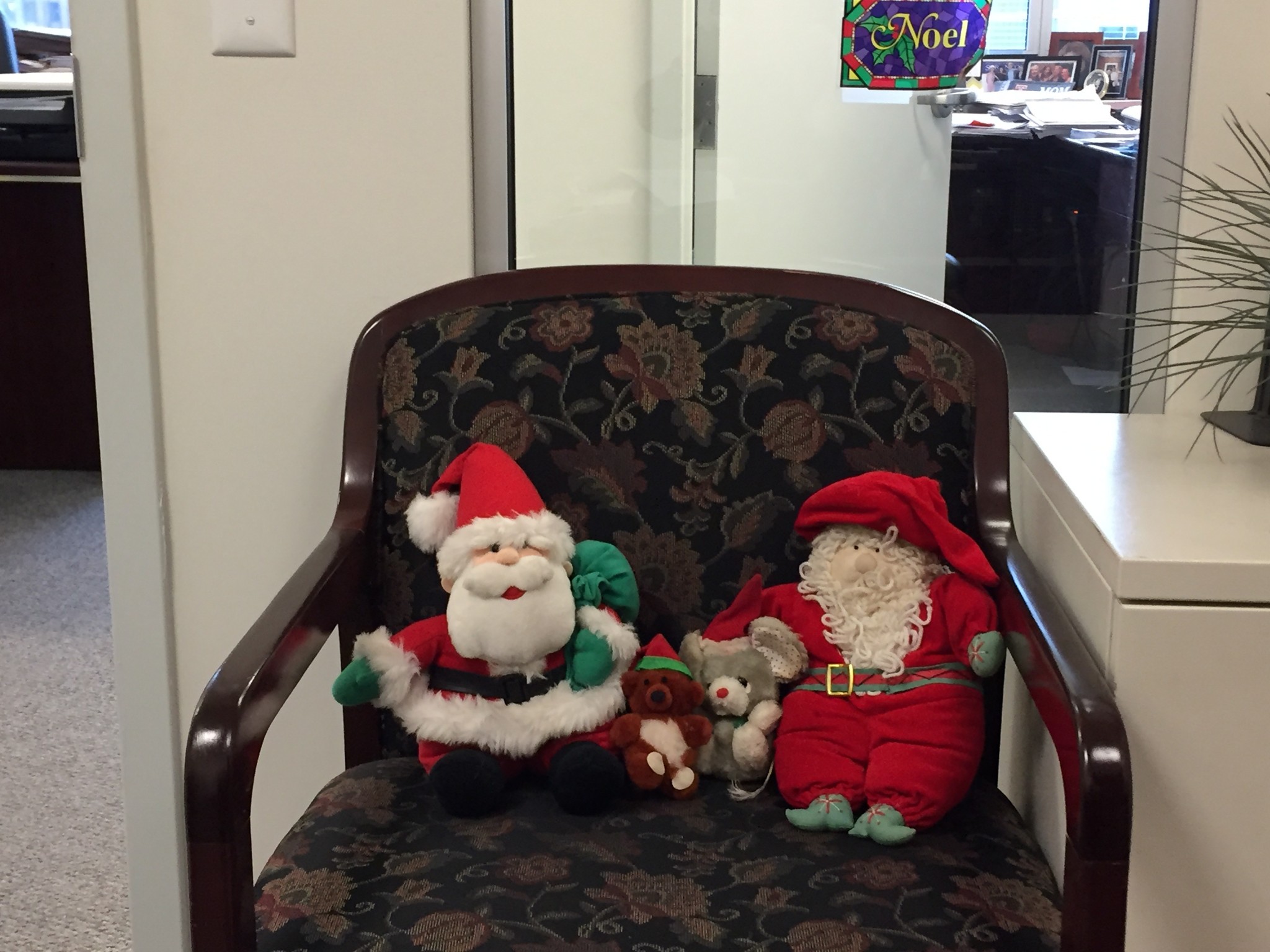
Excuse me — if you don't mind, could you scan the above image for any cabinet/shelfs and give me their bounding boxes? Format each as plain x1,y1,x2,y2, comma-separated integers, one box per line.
0,160,103,474
1003,400,1270,952
944,139,1102,314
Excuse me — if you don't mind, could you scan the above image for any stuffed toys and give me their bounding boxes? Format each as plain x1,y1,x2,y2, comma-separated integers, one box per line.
619,618,809,803
707,469,1006,842
331,441,635,806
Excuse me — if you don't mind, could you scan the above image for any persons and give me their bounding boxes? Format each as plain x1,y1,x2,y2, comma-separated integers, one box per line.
1089,64,1119,95
986,62,1023,92
1028,63,1070,83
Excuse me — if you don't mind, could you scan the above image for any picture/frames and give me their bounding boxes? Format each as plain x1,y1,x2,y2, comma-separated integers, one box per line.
1127,30,1147,99
1022,54,1083,90
1086,43,1134,101
981,53,1039,79
1047,31,1104,91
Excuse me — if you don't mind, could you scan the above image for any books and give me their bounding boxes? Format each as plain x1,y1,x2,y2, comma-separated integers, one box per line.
951,84,1139,145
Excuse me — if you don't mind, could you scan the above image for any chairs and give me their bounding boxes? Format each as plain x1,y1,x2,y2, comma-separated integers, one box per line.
177,263,1135,952
